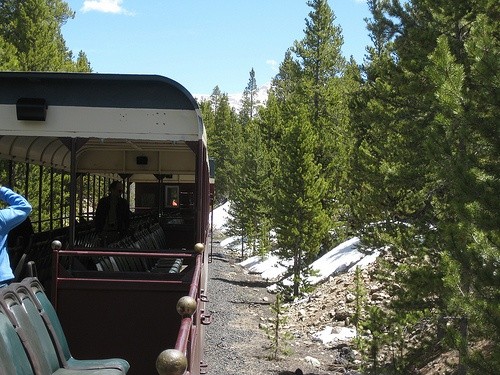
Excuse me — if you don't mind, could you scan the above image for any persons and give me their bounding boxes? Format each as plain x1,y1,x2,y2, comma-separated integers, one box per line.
96,180,129,240
0,184,32,288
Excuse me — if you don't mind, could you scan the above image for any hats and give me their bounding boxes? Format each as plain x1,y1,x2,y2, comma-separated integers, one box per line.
111,181,123,195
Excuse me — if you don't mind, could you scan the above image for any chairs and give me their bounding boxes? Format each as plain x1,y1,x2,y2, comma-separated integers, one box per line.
0,277,130,375
96,210,187,273
